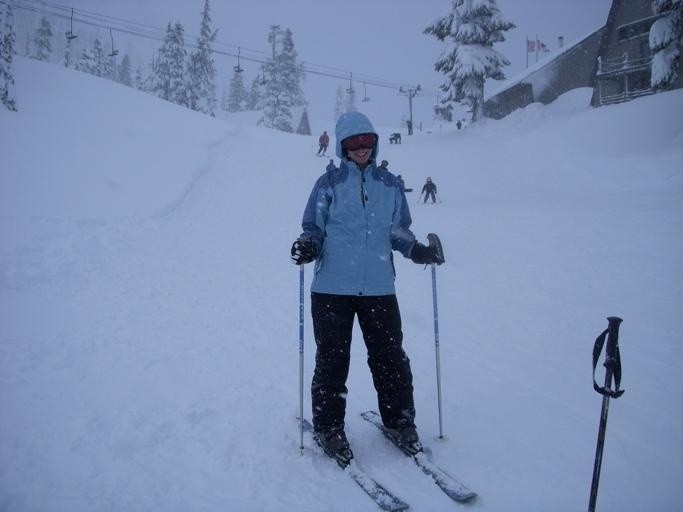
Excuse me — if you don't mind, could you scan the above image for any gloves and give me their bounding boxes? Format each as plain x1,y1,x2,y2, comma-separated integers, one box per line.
290,242,311,266
413,241,441,265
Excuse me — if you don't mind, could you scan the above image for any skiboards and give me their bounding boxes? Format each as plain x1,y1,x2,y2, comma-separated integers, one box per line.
296,411,479,511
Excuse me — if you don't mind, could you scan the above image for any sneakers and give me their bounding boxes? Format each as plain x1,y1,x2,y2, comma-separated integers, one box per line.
315,426,356,468
382,419,426,457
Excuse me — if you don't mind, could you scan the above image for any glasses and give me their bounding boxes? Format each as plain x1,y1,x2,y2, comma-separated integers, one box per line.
344,135,377,149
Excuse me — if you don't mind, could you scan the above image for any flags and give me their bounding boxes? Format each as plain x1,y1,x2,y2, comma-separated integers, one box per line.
537,38,550,53
525,39,535,53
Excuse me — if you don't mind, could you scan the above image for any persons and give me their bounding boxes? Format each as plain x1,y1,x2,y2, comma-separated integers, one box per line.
395,173,405,189
287,109,446,466
315,130,329,155
379,159,388,169
455,119,462,130
386,132,402,145
325,158,336,172
420,176,438,204
405,120,411,136
